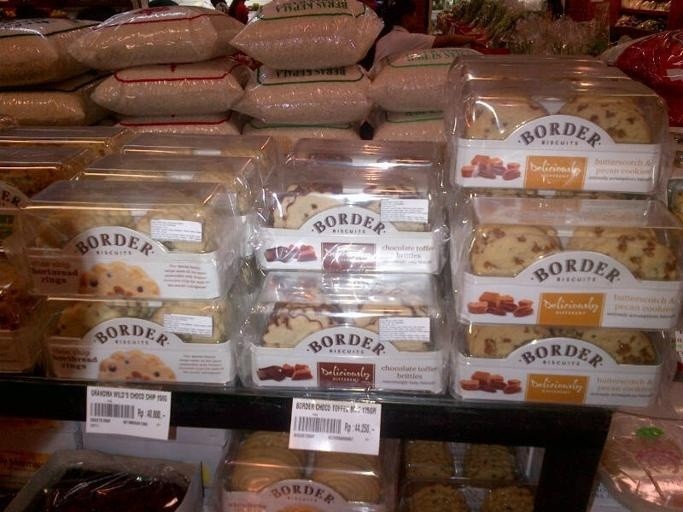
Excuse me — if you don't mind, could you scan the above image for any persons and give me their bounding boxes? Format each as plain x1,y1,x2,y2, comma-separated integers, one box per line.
227,1,248,25
209,0,227,15
366,0,488,83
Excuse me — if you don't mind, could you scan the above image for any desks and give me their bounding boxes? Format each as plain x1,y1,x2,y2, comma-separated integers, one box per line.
0,367,614,512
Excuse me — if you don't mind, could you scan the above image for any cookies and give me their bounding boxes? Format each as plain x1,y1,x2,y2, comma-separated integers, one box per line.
0,92,683,406
0,411,683,512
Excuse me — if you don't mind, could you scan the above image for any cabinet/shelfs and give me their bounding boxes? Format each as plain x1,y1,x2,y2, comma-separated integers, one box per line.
605,0,682,45
